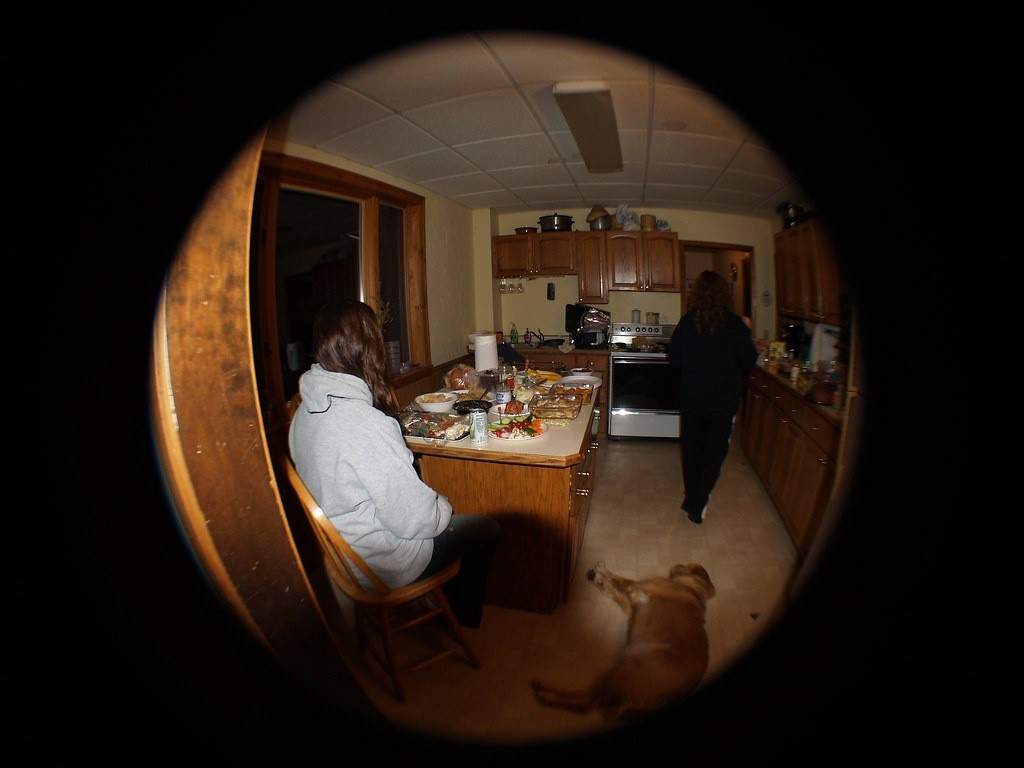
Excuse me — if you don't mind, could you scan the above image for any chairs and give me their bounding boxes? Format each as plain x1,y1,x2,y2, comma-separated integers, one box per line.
272,446,483,706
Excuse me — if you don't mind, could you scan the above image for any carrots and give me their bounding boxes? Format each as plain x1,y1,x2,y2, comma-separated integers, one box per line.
527,420,543,436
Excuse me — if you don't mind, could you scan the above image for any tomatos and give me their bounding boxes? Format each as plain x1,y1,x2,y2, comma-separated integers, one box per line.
494,427,509,438
509,420,528,431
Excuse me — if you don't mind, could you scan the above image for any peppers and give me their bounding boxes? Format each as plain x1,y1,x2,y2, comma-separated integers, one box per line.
523,427,534,436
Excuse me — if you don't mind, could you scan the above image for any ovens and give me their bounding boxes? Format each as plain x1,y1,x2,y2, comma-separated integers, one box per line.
608,351,736,446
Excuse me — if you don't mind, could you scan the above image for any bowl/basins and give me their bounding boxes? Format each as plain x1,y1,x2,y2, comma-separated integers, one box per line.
571,367,593,374
640,215,657,227
414,392,457,412
549,382,594,406
528,393,581,419
587,216,612,230
468,331,487,343
515,227,538,234
643,226,655,231
454,400,493,416
812,381,835,403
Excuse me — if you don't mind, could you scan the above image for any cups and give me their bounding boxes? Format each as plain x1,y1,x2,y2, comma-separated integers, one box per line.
287,343,299,371
631,308,641,323
478,368,497,390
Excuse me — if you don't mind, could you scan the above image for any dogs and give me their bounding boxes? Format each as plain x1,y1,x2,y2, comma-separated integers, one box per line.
528,563,716,725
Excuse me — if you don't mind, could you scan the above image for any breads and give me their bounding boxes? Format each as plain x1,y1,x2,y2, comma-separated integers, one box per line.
450,367,480,390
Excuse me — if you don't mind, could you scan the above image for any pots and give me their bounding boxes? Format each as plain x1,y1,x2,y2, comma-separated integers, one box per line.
531,328,565,349
537,213,575,232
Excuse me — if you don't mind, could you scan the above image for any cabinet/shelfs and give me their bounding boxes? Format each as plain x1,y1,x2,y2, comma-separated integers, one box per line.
773,218,841,325
577,231,609,305
740,350,846,557
509,340,608,441
402,364,602,612
606,230,681,293
491,232,577,280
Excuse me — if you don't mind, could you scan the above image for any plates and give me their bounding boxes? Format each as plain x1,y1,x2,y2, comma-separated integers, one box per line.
558,375,603,389
488,404,533,416
448,390,497,402
392,409,470,443
488,415,549,439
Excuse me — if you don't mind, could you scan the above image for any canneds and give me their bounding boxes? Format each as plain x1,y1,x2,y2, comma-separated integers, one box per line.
790,364,800,382
469,408,488,445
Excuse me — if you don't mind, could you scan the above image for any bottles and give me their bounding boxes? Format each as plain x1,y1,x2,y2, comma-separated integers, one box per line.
647,312,659,324
497,365,512,402
784,349,845,409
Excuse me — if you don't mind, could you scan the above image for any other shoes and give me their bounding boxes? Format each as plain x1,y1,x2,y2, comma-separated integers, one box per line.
681,503,703,523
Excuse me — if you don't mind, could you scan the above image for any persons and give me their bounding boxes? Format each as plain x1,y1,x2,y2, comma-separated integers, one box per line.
667,270,760,525
286,298,501,659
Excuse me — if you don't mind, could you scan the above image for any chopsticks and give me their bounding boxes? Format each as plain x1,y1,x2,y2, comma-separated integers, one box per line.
478,385,492,400
